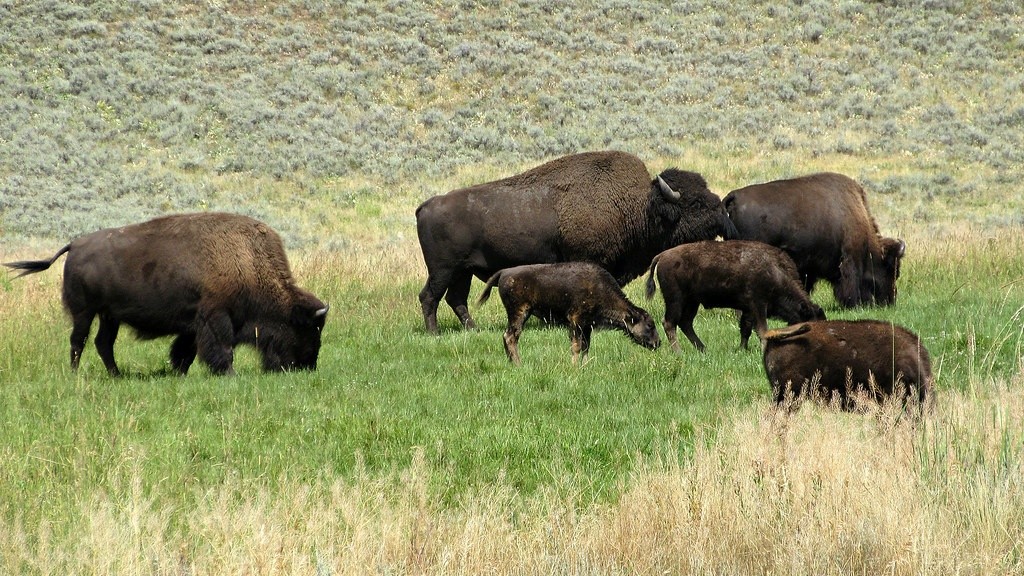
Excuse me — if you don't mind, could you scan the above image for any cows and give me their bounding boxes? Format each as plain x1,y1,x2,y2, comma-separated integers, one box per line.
756,320,933,408
0,210,330,374
414,148,905,368
